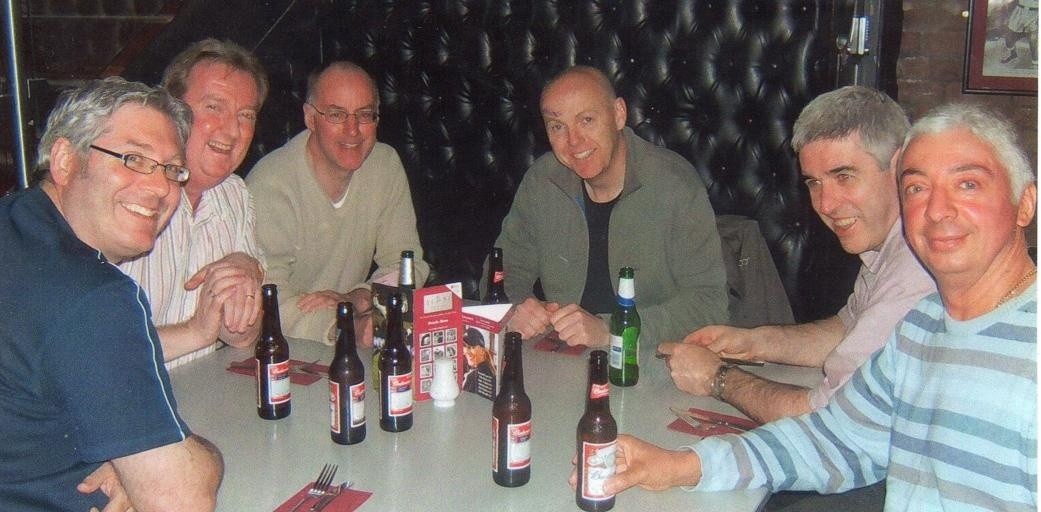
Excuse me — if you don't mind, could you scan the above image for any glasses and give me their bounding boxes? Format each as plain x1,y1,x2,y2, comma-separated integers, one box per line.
90,144,189,184
310,104,379,124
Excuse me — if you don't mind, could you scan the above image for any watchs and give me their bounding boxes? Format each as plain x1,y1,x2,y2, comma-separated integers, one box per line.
708,363,734,403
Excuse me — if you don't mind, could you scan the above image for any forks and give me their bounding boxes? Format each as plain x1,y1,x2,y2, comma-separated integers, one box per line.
287,463,339,512
682,415,747,433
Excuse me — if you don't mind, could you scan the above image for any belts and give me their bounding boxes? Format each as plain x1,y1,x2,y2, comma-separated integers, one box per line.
1018,3,1038,11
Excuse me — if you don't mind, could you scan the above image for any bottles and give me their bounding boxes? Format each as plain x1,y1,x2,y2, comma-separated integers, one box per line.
255,284,291,420
481,248,509,305
328,302,366,445
398,250,417,345
378,293,412,432
492,331,532,488
609,267,639,386
576,350,617,512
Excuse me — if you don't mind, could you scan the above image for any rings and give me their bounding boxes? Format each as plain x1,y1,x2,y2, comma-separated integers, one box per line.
245,293,256,301
209,289,218,299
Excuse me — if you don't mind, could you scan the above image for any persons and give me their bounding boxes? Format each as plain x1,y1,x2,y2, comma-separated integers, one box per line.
113,37,273,378
1000,0,1038,65
0,70,227,512
566,98,1037,510
654,84,943,428
477,64,728,359
241,57,431,352
460,327,495,402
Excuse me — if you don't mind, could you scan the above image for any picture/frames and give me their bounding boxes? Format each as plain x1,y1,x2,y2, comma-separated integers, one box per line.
961,0,1038,98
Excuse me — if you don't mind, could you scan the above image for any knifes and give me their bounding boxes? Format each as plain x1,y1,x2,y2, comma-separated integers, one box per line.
654,354,765,368
669,406,752,432
308,480,355,512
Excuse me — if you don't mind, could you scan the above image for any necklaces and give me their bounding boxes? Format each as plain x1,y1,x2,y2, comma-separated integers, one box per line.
990,268,1036,316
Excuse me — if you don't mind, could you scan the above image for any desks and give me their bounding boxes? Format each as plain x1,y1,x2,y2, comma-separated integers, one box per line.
167,299,826,512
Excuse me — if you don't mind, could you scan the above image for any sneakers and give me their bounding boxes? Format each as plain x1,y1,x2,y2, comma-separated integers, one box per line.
1000,48,1018,63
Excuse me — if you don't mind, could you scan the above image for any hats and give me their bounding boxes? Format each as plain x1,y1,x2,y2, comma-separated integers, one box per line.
462,329,485,348
